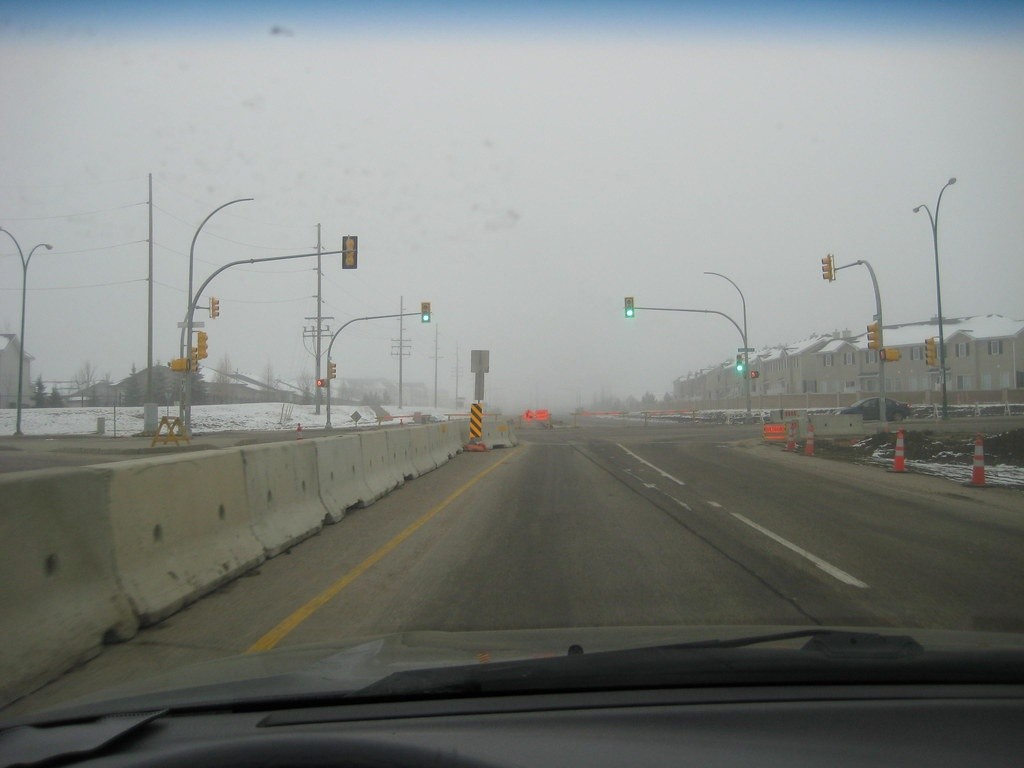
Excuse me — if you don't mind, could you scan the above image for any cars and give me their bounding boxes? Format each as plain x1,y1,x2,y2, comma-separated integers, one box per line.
830,396,911,422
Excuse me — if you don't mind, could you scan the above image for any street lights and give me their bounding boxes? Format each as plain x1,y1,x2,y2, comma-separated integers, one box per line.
1,228,54,436
913,178,957,418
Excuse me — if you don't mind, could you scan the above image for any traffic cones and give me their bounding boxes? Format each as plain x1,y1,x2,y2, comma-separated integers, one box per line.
782,422,797,453
799,425,816,456
885,429,911,473
962,439,994,487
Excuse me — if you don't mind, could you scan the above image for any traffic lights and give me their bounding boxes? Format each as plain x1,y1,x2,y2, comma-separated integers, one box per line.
737,355,742,372
212,298,219,319
191,348,197,372
625,297,634,318
329,362,336,379
867,323,880,349
822,254,832,282
751,371,759,378
925,338,936,366
317,379,324,387
198,332,208,359
879,349,899,361
422,303,430,323
